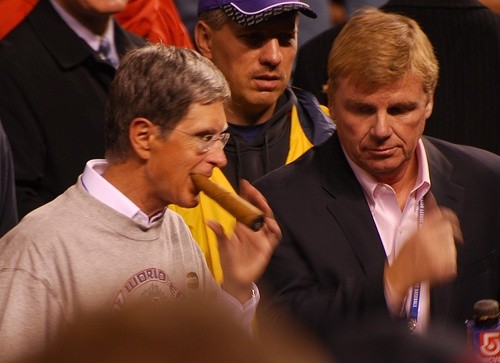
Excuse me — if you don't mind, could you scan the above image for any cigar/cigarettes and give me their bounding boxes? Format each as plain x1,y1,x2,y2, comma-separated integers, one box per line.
189,169,265,233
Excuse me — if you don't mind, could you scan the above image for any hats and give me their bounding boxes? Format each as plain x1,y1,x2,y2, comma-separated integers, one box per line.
198,0,318,27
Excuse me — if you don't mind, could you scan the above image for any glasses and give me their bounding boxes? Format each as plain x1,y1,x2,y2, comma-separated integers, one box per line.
164,125,230,152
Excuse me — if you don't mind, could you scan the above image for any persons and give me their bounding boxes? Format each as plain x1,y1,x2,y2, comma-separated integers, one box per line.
0,1,499,363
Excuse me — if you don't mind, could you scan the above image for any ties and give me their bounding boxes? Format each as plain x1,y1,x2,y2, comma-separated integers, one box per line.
100,40,119,69
